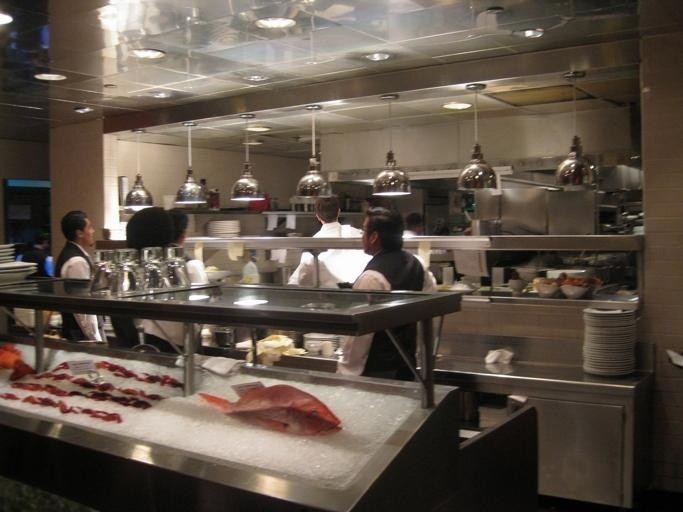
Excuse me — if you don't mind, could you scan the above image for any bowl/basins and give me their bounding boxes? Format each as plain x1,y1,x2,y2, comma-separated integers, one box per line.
207,325,237,348
508,278,523,292
533,282,588,300
203,270,230,282
305,341,321,353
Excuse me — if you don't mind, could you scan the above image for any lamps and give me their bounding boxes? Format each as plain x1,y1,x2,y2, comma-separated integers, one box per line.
175,122,207,204
556,72,595,185
296,105,332,198
458,83,497,192
231,114,265,201
129,129,154,207
371,93,412,198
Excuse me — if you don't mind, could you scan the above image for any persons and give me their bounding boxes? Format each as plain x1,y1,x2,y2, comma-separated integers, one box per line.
283,197,375,293
52,206,106,341
144,209,208,356
399,213,425,239
13,230,53,280
334,204,438,380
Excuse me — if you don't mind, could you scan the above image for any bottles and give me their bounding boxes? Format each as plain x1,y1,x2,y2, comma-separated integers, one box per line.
242,257,258,284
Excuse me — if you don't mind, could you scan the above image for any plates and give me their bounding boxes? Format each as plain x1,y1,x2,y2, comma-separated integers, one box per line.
12,308,63,330
438,289,474,294
579,307,641,376
102,315,114,331
207,220,241,237
302,333,339,350
0,242,39,280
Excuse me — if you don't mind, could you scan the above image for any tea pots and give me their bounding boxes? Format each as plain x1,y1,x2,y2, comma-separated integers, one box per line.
90,258,190,295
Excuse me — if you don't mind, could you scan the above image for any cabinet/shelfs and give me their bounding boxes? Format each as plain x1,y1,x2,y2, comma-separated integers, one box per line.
507,386,635,509
0,283,461,512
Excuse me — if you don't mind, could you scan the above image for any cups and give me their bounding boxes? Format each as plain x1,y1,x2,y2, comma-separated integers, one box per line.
117,175,129,206
491,267,504,287
186,259,210,285
442,267,453,285
